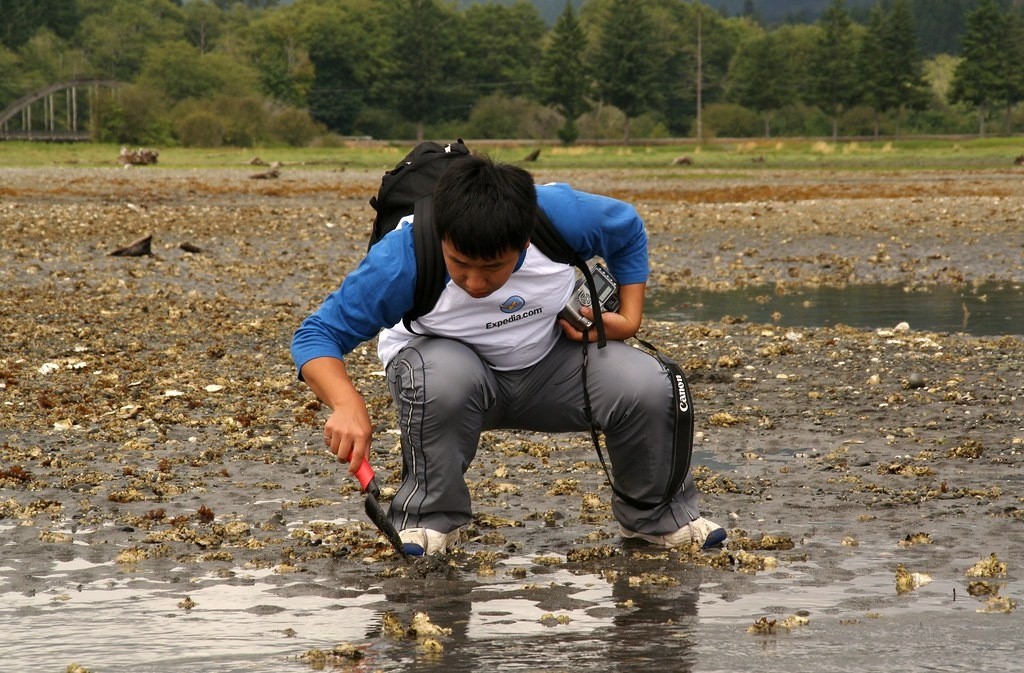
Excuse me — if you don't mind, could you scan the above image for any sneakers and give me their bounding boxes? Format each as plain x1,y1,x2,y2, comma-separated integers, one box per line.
618,514,728,555
394,526,462,560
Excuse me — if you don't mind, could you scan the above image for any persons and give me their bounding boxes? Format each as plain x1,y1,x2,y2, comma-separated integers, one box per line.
290,158,728,556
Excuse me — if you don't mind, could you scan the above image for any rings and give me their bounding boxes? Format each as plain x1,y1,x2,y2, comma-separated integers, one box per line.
323,436,331,439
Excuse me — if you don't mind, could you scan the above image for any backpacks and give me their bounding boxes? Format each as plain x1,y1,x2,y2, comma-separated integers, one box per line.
365,140,584,322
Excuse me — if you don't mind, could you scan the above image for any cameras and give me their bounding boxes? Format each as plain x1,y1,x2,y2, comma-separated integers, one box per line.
556,262,620,333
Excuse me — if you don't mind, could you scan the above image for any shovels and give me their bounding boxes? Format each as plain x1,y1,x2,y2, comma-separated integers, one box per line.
347,448,409,562
